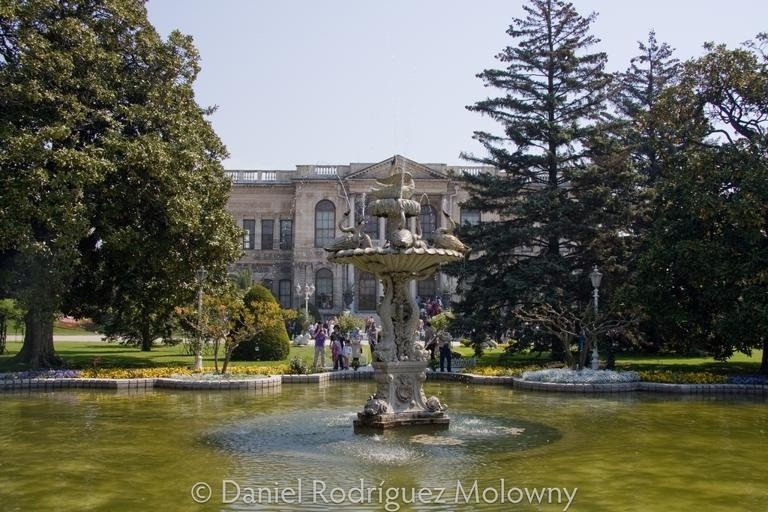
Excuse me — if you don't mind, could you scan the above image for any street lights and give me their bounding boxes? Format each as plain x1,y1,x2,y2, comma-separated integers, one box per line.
194,264,210,372
296,282,316,334
588,265,604,370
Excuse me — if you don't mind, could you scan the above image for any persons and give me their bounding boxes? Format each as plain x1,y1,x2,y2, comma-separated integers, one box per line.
287,296,452,372
